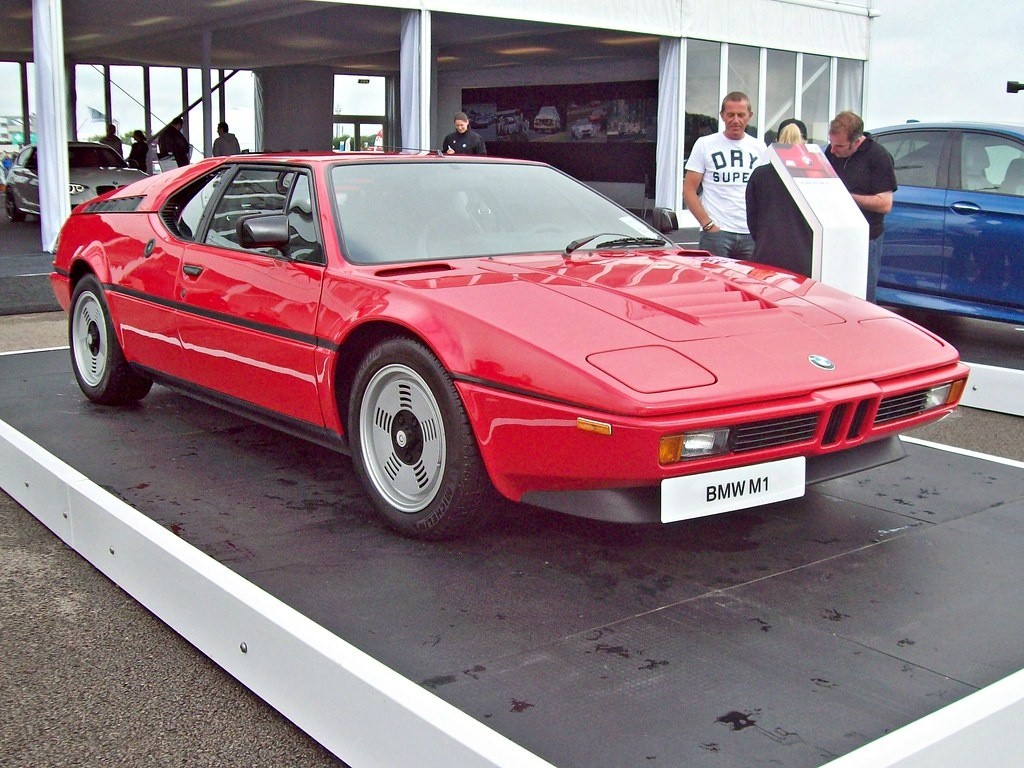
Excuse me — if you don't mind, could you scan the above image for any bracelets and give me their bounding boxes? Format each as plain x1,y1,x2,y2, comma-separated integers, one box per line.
702,222,714,231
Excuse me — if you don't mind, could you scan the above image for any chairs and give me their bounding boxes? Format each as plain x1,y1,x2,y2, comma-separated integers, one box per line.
909,159,937,188
963,144,998,193
418,202,484,258
1000,157,1024,195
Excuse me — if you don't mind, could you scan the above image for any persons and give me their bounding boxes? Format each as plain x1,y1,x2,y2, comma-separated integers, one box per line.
128,130,149,173
819,111,898,304
158,118,190,167
442,112,487,157
212,122,240,157
0,150,12,178
745,118,813,278
684,91,770,260
100,125,123,157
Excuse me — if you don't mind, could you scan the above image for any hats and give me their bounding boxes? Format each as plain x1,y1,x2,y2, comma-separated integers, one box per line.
776,118,807,141
